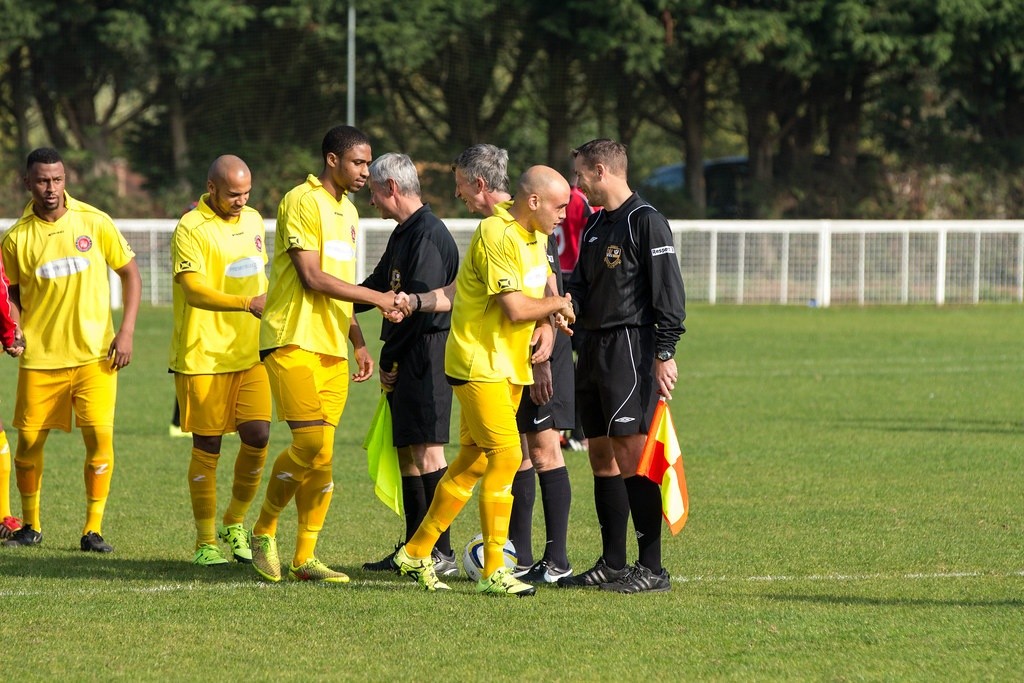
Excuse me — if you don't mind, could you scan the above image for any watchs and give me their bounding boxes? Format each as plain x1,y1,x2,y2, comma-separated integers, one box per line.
655,351,673,362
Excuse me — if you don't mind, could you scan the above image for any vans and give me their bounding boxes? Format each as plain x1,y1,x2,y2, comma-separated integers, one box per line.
641,153,891,220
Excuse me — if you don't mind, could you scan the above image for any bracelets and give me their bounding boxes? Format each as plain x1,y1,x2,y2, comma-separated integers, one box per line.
413,293,422,311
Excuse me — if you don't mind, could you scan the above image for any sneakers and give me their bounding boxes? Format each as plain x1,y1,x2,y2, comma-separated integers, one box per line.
392,545,452,593
286,557,352,583
2,523,44,548
216,520,254,564
0,516,23,539
554,555,631,589
245,521,284,581
521,560,576,589
474,568,536,597
191,542,232,567
598,560,671,594
80,530,112,552
427,547,462,580
512,564,536,581
361,539,410,575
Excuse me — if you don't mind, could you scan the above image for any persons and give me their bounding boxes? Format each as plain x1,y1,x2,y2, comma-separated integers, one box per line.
0,147,142,553
381,144,574,585
247,125,412,583
0,245,27,540
353,152,459,581
390,164,576,599
167,154,272,567
555,138,687,595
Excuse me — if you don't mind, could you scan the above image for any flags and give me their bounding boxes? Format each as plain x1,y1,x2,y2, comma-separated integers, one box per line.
362,393,405,521
636,399,689,536
